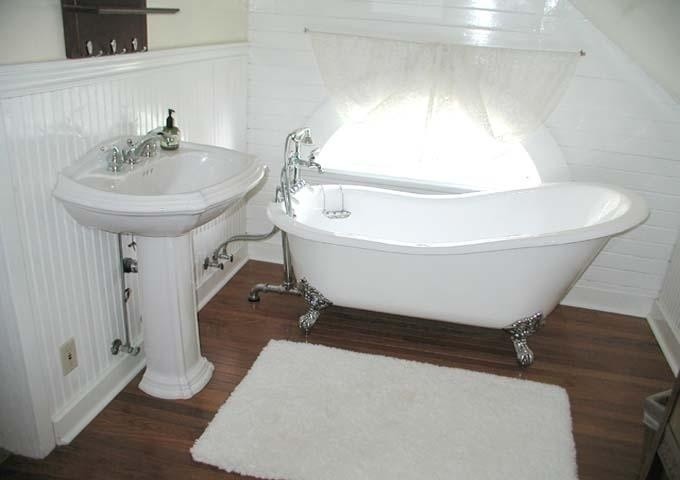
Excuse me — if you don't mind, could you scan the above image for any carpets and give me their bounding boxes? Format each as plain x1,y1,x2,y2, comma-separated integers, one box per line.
189,338,579,479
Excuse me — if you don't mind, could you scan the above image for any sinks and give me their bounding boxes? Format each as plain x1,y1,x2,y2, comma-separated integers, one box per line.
49,135,271,236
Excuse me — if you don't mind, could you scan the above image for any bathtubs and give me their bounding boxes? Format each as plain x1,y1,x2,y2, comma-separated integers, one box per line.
265,180,652,328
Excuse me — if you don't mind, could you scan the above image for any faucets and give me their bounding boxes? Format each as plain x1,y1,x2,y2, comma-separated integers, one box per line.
277,147,326,187
121,132,180,163
